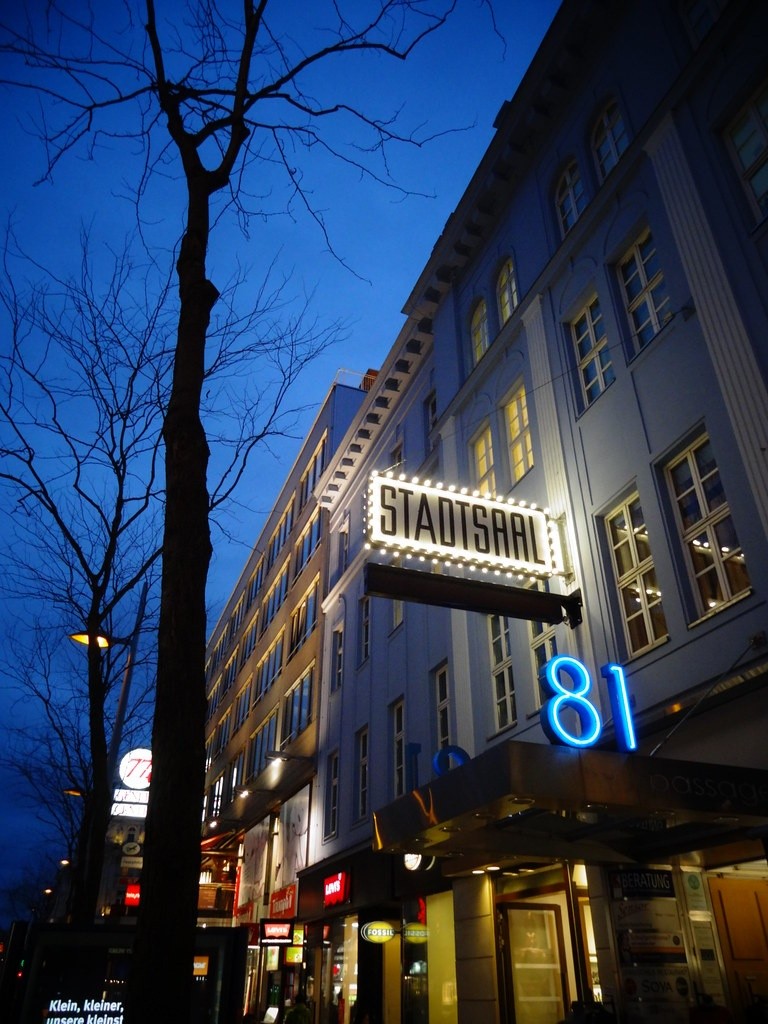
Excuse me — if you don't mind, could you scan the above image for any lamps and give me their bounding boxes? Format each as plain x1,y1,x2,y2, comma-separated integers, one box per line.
265,751,314,760
235,786,277,796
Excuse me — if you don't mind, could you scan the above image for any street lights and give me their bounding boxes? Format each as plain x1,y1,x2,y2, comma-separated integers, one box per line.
68,631,138,787
